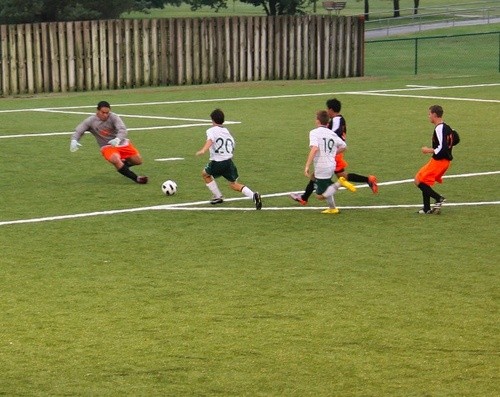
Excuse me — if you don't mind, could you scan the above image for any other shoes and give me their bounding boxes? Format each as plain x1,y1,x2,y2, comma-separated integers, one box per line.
137,175,148,184
289,192,307,206
252,192,262,211
321,208,340,214
415,208,432,215
368,176,378,195
338,177,356,192
210,197,223,205
433,196,446,211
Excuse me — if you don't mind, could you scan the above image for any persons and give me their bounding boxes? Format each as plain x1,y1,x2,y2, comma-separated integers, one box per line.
195,108,262,210
414,105,460,214
304,110,356,214
70,101,148,184
289,98,378,205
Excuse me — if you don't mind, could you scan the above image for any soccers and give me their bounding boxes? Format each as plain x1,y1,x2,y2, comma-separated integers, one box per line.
162,180,177,196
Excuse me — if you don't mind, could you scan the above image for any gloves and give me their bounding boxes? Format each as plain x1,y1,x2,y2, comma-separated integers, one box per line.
70,139,83,152
107,137,121,147
